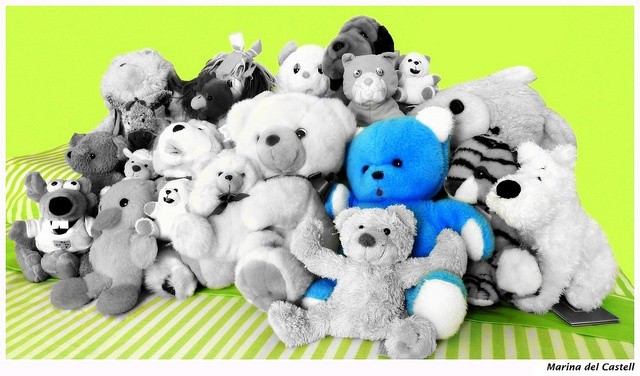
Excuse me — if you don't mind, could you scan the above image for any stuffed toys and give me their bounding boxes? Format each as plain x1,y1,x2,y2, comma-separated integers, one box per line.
13,17,616,360
